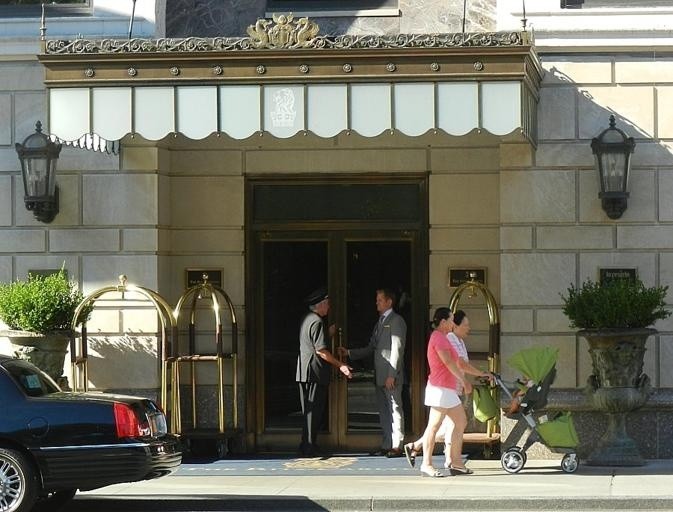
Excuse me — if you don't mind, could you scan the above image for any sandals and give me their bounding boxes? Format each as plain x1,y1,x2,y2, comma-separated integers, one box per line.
404,442,473,477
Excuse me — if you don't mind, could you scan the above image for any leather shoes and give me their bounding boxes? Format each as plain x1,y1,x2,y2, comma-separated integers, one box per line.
297,445,330,458
369,448,403,458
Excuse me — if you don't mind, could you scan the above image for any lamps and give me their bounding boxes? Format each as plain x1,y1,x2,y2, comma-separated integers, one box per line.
13,121,59,223
590,114,636,219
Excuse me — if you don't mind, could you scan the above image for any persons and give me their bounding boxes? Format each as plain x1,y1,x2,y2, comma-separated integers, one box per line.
403,310,495,470
336,291,409,460
290,291,354,460
420,306,473,478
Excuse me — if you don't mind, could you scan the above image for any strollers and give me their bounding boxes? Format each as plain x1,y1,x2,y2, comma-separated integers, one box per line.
472,363,580,472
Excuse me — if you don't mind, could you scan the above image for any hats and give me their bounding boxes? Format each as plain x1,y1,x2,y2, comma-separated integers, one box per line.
308,289,328,305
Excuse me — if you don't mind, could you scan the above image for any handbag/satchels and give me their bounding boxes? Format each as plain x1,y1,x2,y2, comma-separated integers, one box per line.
473,377,496,423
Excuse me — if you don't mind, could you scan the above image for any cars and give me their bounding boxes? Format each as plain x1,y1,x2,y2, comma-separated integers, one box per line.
0,355,182,510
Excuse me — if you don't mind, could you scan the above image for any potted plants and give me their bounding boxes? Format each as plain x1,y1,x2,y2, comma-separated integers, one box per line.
0,260,97,392
558,272,672,467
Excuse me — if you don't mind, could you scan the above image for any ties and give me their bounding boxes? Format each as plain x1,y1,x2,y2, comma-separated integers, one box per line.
376,316,384,336
